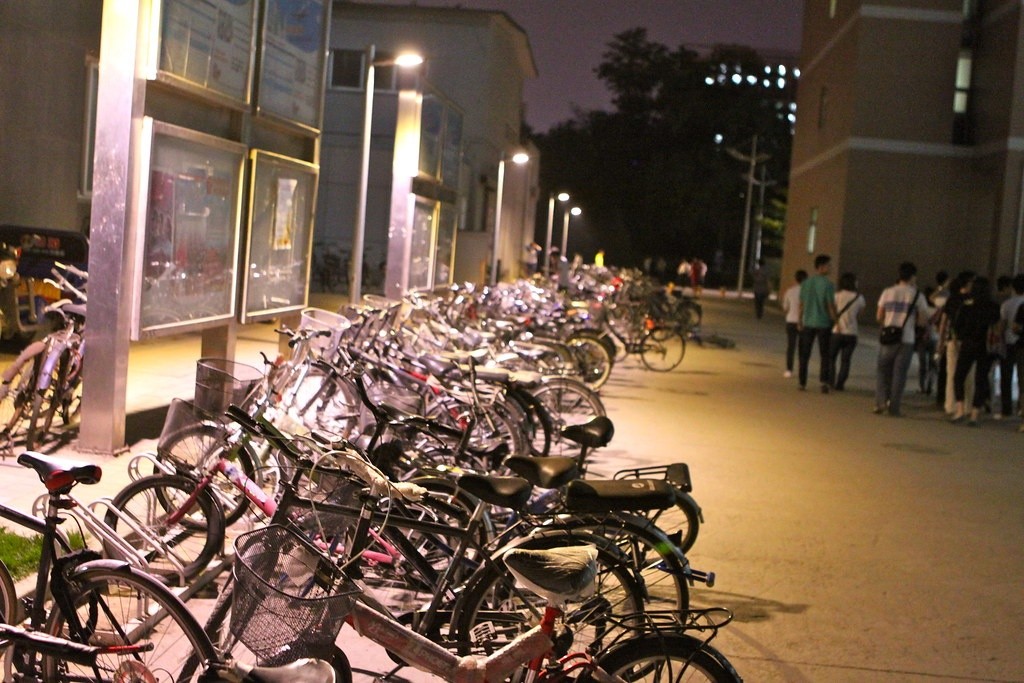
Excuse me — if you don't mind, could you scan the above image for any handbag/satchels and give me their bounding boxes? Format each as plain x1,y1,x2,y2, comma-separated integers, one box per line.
880,326,902,345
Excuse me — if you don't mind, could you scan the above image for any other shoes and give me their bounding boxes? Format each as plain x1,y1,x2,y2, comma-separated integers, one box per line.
967,414,979,427
784,370,792,378
822,384,829,394
993,412,1004,421
952,413,965,425
838,383,844,391
889,403,898,415
874,403,887,414
798,384,805,391
936,395,944,406
983,399,993,414
916,388,933,396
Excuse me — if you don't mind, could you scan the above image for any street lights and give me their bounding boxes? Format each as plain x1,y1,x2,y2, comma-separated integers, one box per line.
492,154,530,286
348,52,426,306
542,192,570,277
561,207,583,259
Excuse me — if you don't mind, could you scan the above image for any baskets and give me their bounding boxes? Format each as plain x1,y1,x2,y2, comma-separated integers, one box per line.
277,439,362,537
159,397,225,470
193,358,265,424
300,307,352,360
363,294,403,336
359,385,424,435
230,523,364,667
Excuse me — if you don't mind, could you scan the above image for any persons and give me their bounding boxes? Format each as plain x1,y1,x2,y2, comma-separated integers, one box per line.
782,254,866,394
65,215,91,323
668,259,730,301
751,258,773,319
873,262,1024,428
523,242,606,294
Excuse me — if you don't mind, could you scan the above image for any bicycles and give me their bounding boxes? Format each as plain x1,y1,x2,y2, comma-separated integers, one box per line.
0,242,746,683
